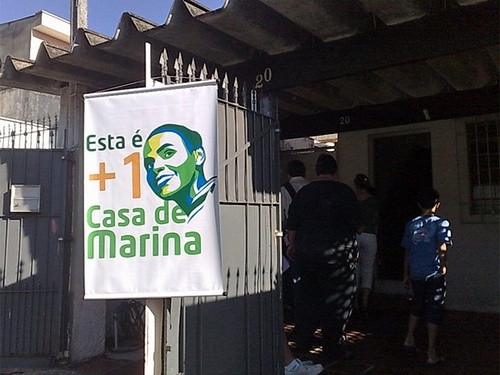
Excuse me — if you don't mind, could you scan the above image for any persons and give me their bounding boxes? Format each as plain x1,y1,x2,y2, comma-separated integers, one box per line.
286,154,366,363
354,170,378,315
284,330,323,375
280,160,311,246
400,190,453,366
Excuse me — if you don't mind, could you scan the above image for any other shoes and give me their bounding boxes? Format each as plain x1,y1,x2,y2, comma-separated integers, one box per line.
403,343,416,352
423,357,441,366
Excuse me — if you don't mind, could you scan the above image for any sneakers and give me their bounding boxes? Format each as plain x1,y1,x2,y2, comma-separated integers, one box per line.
283,358,323,375
302,360,313,365
